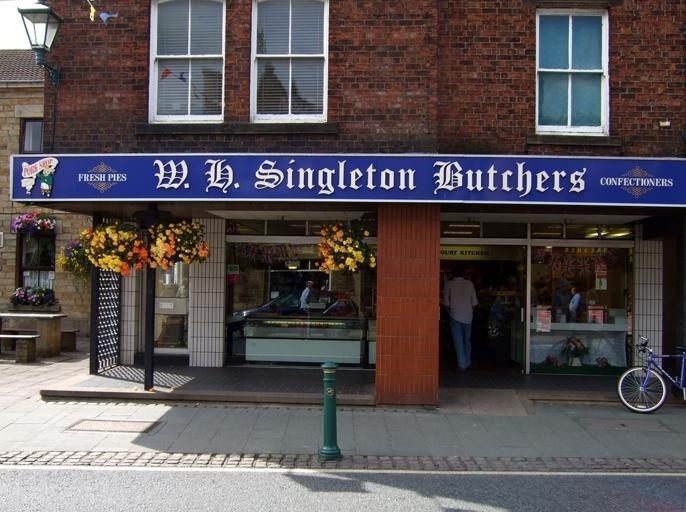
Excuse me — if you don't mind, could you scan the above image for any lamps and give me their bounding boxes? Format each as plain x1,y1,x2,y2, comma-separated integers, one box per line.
17,4,64,89
288,258,298,270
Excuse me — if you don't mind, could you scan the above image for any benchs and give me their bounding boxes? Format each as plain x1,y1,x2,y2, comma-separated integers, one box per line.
1,328,80,351
0,334,40,363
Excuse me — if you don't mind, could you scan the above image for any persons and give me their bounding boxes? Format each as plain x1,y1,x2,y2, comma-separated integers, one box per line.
443,260,481,372
298,279,314,311
552,282,572,306
568,286,583,322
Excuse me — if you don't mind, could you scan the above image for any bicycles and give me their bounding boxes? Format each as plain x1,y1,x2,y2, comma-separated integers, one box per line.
615,337,685,414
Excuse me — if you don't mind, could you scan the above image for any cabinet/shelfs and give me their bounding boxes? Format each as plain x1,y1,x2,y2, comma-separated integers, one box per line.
530,323,628,367
225,313,246,356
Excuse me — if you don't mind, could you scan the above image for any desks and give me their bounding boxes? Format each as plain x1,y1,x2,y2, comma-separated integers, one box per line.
0,313,68,358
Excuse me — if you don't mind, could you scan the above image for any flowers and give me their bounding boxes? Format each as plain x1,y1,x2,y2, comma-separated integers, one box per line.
10,212,56,232
595,356,608,368
541,353,560,368
79,222,148,277
56,227,95,271
10,287,55,305
148,221,210,272
560,342,572,353
318,223,377,274
567,338,589,357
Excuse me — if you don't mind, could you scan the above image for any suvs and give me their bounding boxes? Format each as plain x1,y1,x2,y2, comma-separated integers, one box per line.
236,292,358,320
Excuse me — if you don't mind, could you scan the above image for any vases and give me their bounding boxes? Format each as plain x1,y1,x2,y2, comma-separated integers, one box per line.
28,227,36,237
568,356,572,366
572,358,582,366
